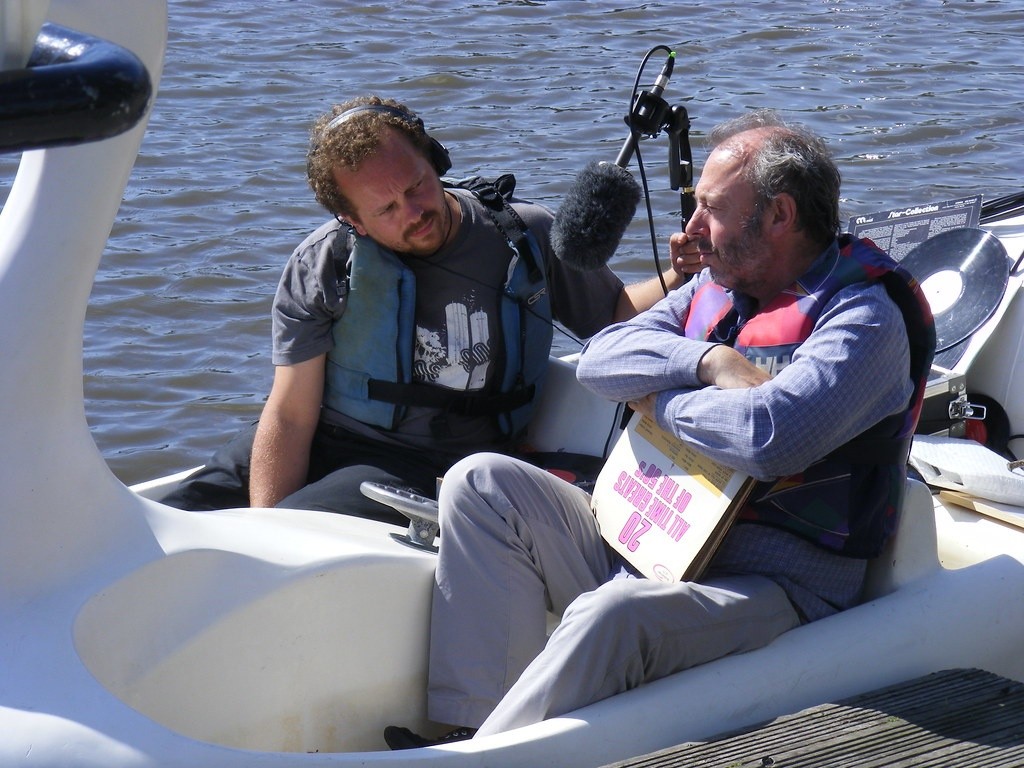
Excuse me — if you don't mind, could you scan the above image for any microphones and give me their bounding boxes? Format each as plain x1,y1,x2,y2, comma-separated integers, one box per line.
550,56,676,273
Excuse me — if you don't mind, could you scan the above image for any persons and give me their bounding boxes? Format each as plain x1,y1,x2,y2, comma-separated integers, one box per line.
383,105,939,751
156,94,713,531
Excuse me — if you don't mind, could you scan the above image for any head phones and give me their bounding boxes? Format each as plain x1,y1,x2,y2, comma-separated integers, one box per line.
307,105,453,226
